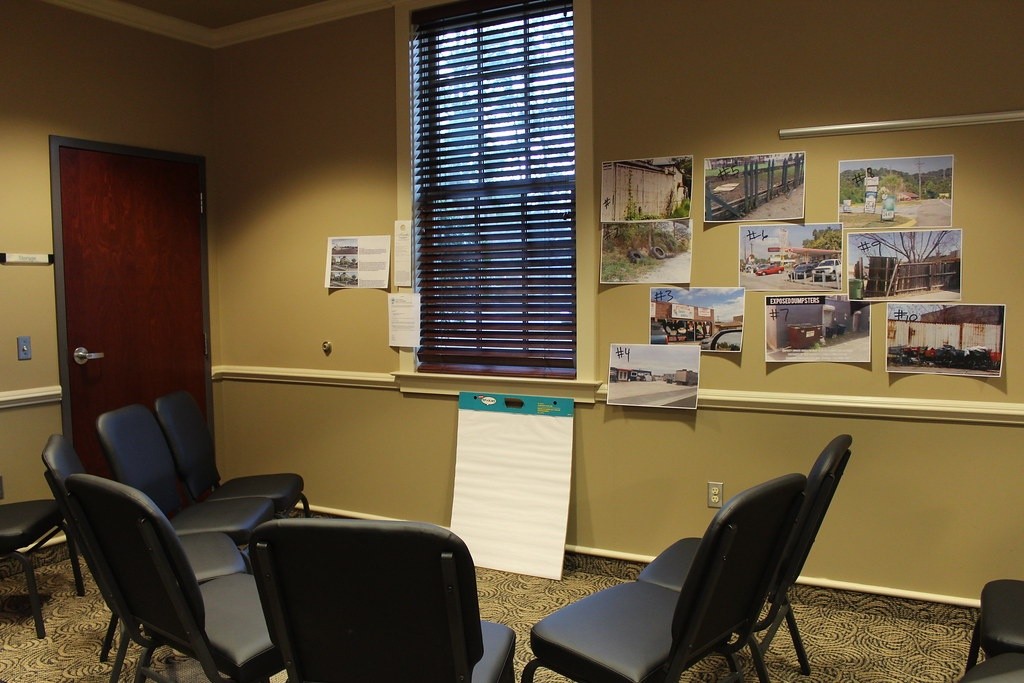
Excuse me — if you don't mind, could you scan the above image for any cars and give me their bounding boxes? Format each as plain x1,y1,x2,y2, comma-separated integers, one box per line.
747,258,841,281
651,322,668,345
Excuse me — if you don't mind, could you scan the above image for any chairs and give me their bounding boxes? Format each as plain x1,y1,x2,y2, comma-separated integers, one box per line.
43,434,253,683
63,472,287,683
96,404,276,547
964,579,1024,674
249,520,516,683
956,651,1024,683
0,476,84,641
636,431,854,683
152,391,312,520
520,473,809,683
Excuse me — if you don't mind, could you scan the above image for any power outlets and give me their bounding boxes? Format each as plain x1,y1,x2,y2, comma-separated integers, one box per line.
707,481,724,508
